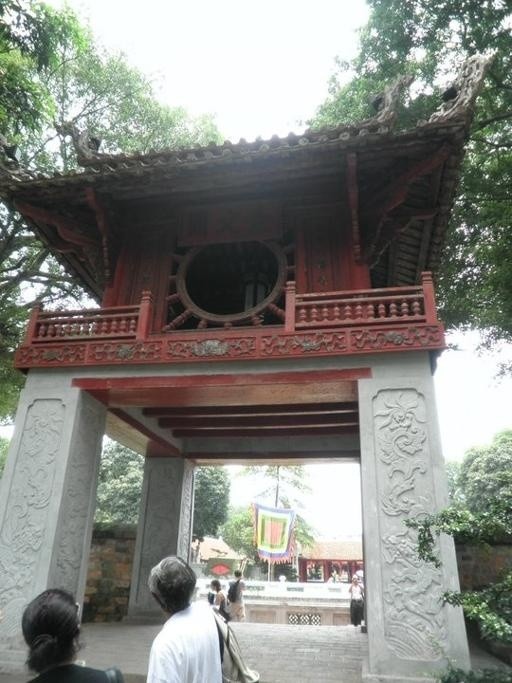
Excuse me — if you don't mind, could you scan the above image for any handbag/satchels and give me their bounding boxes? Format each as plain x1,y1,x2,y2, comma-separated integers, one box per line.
228,581,239,602
222,602,233,621
221,626,259,682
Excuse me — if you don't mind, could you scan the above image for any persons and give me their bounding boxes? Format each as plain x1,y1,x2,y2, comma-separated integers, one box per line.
227,569,246,622
348,575,364,627
209,579,228,624
146,555,227,683
22,588,125,683
